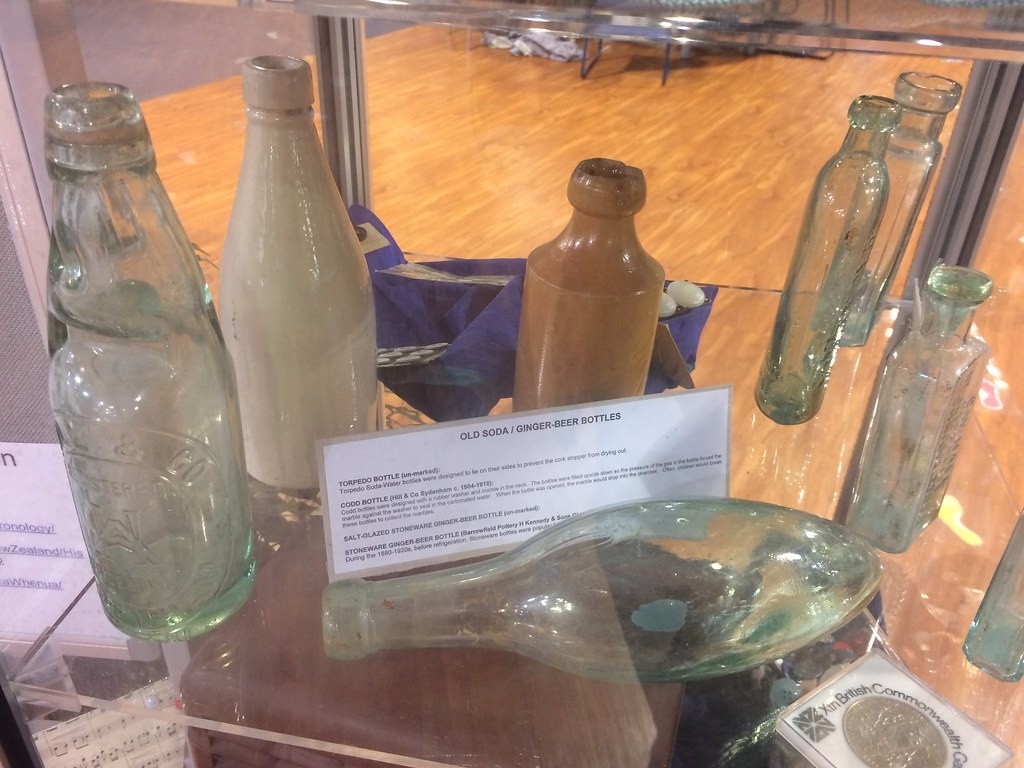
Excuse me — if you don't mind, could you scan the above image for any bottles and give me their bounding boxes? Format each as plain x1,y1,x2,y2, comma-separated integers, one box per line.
813,74,964,348
511,157,663,411
830,266,993,553
323,498,880,682
42,80,255,643
755,91,901,423
217,55,378,490
963,507,1024,686
